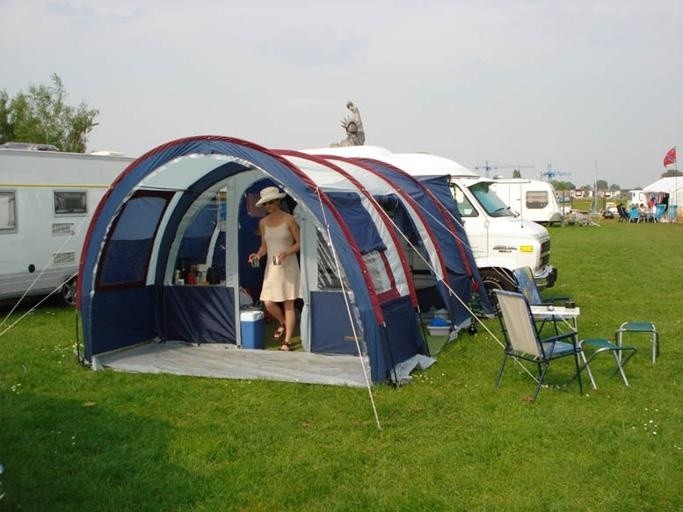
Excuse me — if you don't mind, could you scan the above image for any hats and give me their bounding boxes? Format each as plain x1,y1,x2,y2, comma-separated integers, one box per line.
255,186,288,207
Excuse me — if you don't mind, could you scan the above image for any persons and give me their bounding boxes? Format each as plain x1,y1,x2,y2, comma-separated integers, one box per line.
328,100,365,147
247,186,299,352
616,197,658,223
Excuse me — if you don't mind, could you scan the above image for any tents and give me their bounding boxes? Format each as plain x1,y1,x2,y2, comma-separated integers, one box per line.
72,133,494,387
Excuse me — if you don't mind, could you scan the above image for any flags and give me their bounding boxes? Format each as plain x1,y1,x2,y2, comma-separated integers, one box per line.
663,147,675,168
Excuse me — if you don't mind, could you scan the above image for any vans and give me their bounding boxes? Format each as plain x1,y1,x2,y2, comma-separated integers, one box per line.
293,146,558,318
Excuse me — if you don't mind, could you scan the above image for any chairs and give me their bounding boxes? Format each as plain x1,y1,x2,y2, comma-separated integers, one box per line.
616,204,680,224
488,267,585,404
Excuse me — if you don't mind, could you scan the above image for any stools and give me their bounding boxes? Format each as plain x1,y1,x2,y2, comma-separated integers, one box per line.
578,322,660,388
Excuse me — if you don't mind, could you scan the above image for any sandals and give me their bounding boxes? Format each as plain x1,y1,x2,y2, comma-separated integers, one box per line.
273,323,292,352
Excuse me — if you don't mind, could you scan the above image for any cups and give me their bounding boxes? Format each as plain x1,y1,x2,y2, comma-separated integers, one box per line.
252,256,259,269
566,302,576,308
272,256,282,265
